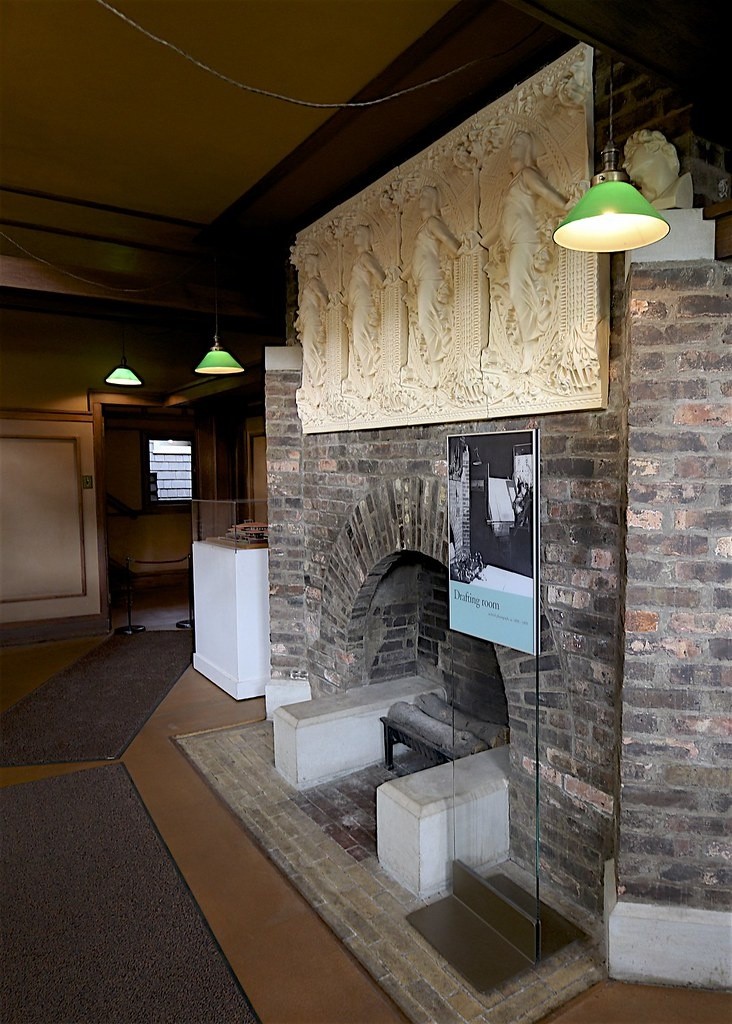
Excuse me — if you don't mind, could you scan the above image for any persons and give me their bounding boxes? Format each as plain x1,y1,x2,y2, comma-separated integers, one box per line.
512,482,532,527
295,252,327,390
480,130,568,374
622,130,693,210
340,225,388,400
399,186,460,390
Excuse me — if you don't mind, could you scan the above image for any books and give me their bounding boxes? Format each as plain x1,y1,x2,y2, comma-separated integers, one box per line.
220,522,268,544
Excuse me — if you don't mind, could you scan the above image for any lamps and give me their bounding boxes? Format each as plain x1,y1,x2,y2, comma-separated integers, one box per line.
103,316,145,388
194,289,245,377
554,44,672,253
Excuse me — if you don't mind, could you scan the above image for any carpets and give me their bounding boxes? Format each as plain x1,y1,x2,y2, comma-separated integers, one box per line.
0,629,196,766
0,760,265,1024
